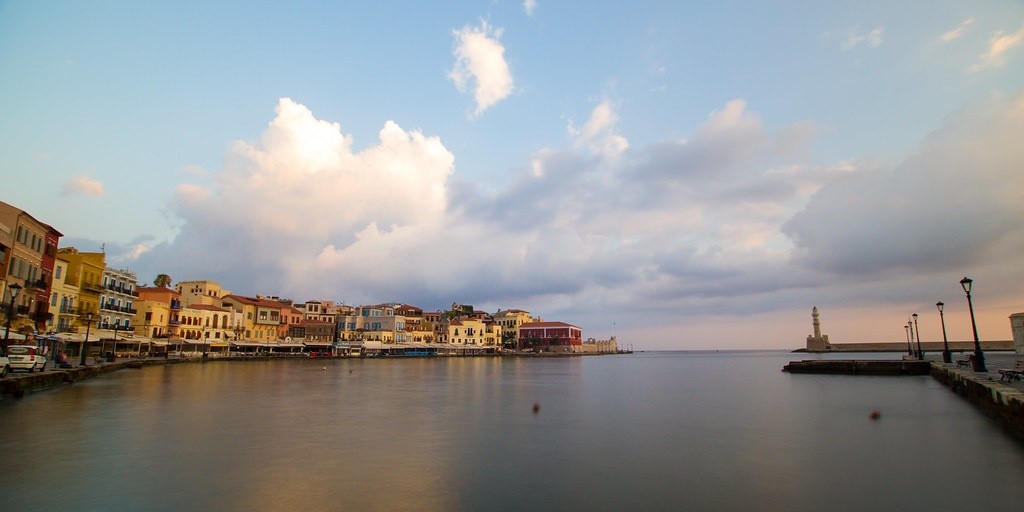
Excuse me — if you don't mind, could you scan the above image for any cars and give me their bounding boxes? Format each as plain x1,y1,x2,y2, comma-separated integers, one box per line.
0,347,9,378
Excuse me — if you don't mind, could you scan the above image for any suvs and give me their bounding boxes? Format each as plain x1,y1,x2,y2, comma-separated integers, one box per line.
8,346,47,372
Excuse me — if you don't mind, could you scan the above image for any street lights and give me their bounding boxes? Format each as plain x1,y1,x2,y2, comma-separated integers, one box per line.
79,310,95,365
112,322,120,358
3,283,23,354
908,321,917,358
203,334,207,360
904,325,912,355
912,313,924,360
960,277,986,372
936,301,953,363
165,329,172,358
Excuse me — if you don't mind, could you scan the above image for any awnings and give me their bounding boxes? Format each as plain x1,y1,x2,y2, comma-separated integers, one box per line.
224,331,236,337
361,344,501,349
231,342,306,347
303,341,332,347
150,338,212,346
36,332,149,344
0,329,26,340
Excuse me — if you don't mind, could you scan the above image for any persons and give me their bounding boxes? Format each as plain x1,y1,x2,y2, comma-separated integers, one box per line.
40,341,48,353
55,349,68,368
